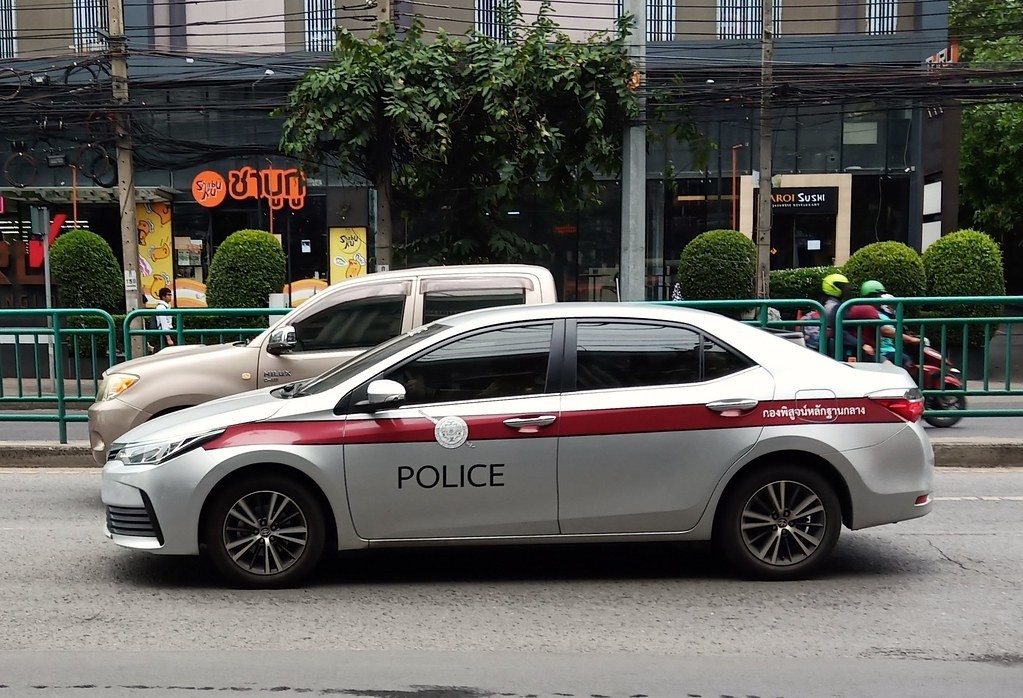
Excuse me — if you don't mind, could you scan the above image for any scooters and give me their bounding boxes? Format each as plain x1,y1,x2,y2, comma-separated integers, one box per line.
842,326,967,429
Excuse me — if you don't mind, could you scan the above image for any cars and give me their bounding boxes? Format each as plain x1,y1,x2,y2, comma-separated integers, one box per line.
98,302,937,591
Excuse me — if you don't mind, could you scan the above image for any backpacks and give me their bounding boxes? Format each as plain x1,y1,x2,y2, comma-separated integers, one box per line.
145,304,167,340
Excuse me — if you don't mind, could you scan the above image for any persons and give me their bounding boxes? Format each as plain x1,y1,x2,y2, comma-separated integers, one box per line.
822,273,875,363
155,288,174,350
878,293,910,367
846,279,931,362
403,353,541,401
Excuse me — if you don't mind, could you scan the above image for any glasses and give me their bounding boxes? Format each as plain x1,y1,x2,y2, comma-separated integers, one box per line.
166,294,171,296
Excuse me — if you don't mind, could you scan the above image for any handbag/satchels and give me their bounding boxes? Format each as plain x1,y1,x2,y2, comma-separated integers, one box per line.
800,311,832,345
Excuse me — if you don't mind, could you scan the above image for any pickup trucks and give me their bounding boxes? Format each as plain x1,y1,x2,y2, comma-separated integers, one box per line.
88,264,804,470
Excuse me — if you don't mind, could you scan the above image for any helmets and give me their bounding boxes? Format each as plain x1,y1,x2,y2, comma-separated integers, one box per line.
861,281,888,296
822,274,850,296
880,294,896,314
